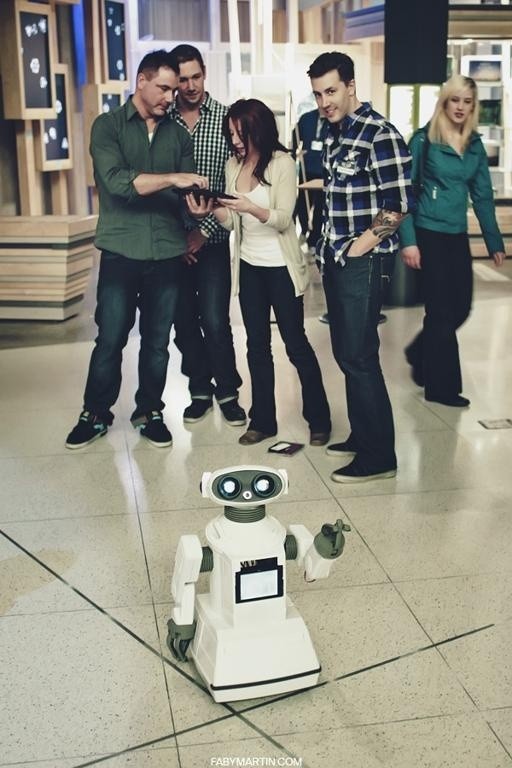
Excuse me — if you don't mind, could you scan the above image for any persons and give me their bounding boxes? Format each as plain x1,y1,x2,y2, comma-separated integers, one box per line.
398,77,505,407
291,109,332,262
166,45,247,427
208,99,333,446
307,52,414,483
64,51,208,449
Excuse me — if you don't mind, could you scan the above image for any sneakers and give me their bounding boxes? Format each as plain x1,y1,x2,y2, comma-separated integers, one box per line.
65,410,114,450
217,396,246,426
326,442,357,455
183,395,214,423
139,409,173,448
331,455,398,483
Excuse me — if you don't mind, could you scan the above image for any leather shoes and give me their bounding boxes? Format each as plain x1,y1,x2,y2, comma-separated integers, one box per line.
239,427,278,446
425,392,471,407
309,430,331,446
403,345,426,387
